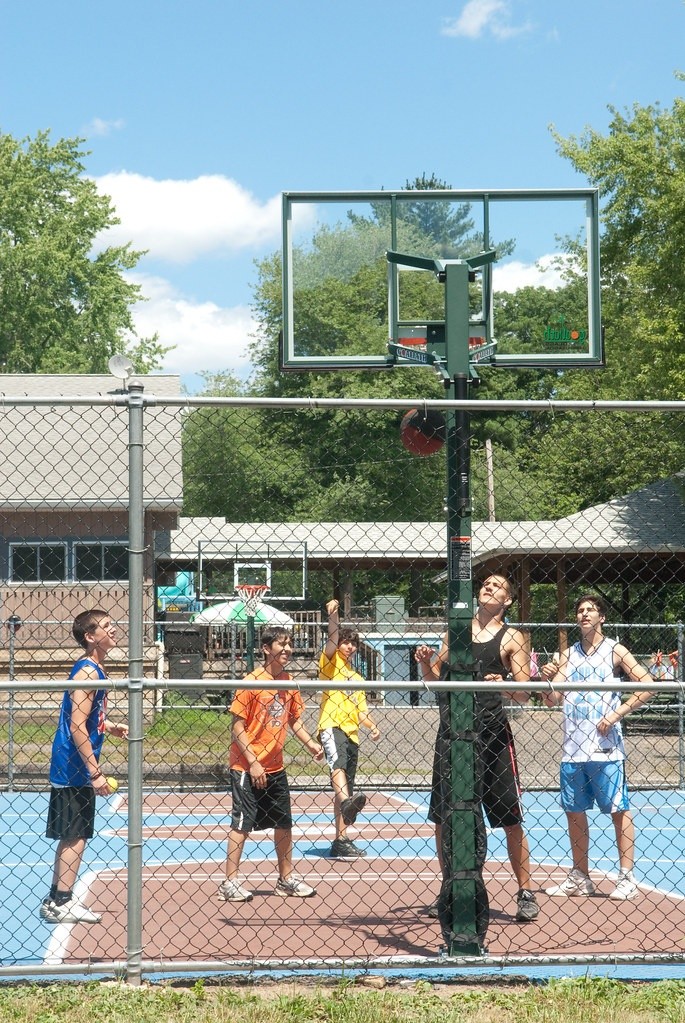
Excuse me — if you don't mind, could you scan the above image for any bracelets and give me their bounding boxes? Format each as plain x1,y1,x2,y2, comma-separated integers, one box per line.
90,770,102,780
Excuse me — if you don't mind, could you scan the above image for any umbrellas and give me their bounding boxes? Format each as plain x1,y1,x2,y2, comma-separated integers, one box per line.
189,601,293,676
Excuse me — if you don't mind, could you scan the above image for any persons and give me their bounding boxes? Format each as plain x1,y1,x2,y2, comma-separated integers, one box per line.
649,650,678,679
40,610,129,923
541,596,656,899
316,599,380,857
218,626,324,901
414,573,538,922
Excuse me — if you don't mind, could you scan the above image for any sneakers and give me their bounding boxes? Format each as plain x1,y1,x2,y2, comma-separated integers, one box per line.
274,876,317,898
330,839,367,856
429,894,441,917
219,877,253,901
40,898,90,918
547,873,594,897
516,895,540,918
344,792,367,824
610,875,639,899
45,894,102,923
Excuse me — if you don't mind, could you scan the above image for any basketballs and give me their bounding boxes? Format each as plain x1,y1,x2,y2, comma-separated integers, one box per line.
400,409,447,455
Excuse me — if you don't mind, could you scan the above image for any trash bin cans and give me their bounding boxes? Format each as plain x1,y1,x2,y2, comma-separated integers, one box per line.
169,654,203,699
